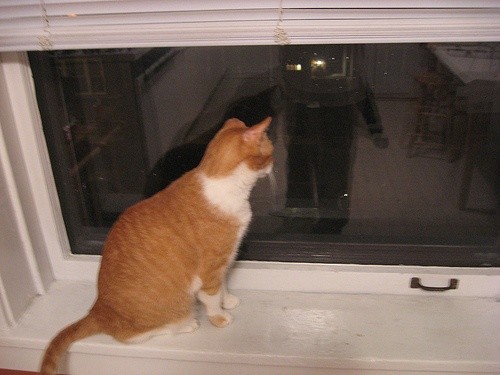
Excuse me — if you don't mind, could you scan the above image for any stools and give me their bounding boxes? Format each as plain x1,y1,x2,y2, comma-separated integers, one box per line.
406,68,496,163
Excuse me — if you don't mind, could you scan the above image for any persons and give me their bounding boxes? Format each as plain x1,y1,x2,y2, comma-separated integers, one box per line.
267,45,389,243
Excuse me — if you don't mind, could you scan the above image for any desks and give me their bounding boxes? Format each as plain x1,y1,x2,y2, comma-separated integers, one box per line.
405,44,500,210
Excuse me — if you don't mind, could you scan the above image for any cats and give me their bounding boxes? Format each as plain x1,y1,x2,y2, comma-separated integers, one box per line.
40,116,278,375
143,84,279,199
